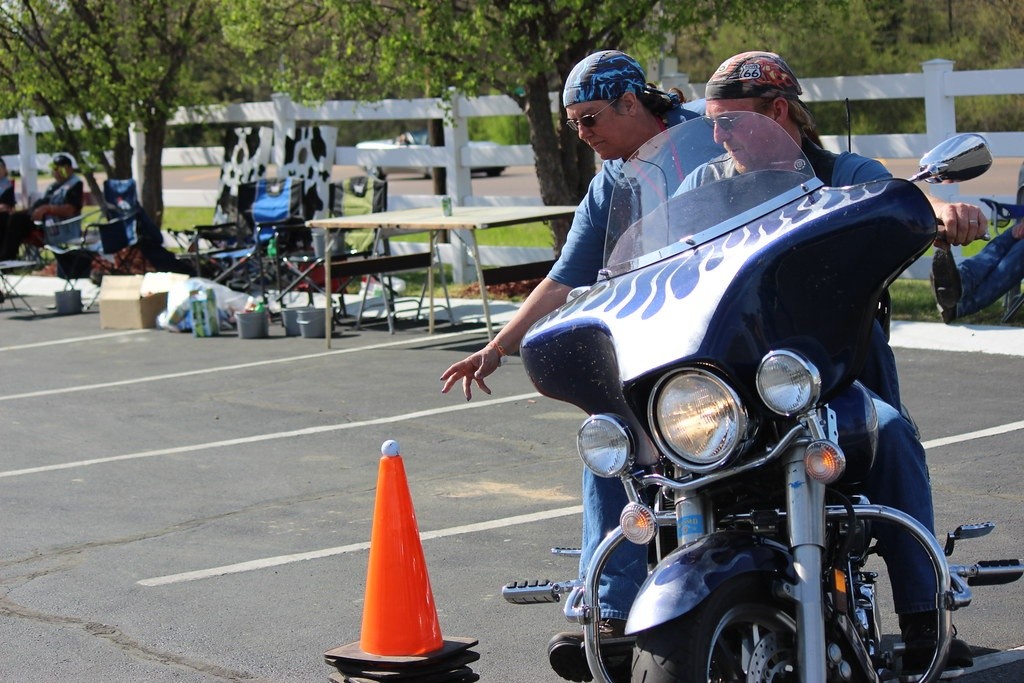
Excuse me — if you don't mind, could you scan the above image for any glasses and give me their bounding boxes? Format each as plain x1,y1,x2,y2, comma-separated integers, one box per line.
703,96,779,131
566,92,626,131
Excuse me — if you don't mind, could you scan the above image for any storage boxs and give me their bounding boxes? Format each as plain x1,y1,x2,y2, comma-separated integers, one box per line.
97,274,168,329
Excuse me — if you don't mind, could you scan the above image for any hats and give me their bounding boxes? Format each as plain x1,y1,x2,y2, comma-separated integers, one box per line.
53,156,72,167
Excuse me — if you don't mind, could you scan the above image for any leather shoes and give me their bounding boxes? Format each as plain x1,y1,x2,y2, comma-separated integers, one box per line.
901,615,973,669
547,618,629,682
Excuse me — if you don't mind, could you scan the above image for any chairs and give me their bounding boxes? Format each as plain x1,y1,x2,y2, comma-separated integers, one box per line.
0,175,456,336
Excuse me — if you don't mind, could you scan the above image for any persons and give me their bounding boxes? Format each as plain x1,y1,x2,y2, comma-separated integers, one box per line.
0,157,19,305
547,52,987,683
0,155,84,259
398,132,411,146
440,50,727,571
932,159,1024,325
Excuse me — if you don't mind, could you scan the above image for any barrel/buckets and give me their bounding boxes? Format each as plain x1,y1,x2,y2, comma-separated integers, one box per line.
296,308,326,338
234,311,269,339
281,306,314,337
55,290,82,316
310,230,344,259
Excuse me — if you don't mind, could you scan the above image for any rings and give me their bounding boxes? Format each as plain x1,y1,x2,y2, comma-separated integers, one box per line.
969,219,978,224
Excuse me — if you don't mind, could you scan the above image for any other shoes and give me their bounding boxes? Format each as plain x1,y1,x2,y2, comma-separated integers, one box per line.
931,245,960,323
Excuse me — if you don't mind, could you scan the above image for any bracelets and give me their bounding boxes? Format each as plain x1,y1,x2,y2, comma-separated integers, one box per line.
489,339,507,367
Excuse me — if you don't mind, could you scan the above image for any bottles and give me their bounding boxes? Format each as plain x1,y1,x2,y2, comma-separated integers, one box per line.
267,240,276,258
117,197,131,210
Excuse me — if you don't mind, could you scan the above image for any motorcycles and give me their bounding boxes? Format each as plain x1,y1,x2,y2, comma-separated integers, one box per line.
498,109,1024,683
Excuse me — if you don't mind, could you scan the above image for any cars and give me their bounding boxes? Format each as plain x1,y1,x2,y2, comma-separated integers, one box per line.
355,128,507,181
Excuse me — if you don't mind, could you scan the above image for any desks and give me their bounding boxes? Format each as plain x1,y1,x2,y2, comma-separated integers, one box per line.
304,204,576,348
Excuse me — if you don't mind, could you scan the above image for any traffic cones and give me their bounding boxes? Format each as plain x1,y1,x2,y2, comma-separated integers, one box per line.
323,439,482,683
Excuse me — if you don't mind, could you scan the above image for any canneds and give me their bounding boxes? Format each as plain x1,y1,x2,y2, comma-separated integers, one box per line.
442,196,452,216
